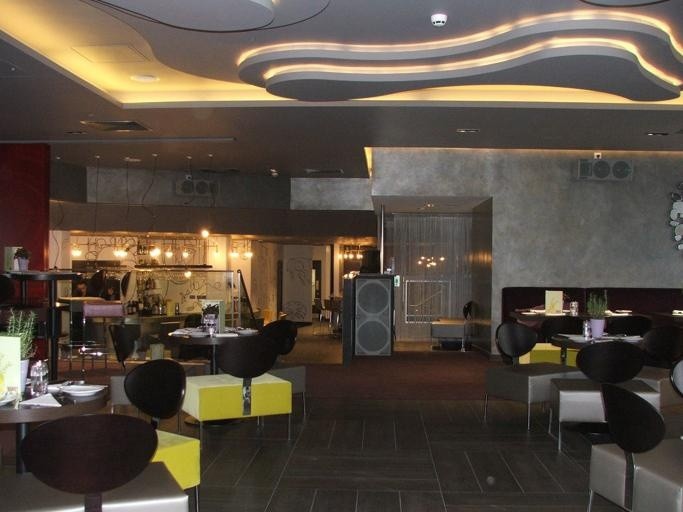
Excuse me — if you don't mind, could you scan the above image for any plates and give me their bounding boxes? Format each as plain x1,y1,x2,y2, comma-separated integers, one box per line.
56,384,106,396
168,329,258,339
566,336,594,344
618,337,644,343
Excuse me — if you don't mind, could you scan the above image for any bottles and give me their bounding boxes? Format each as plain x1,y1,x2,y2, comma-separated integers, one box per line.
125,298,167,315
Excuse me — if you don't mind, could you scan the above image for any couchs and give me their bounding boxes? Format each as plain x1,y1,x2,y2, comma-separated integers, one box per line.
496,287,683,365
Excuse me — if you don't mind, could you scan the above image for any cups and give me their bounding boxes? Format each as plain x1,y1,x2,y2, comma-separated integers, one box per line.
582,320,593,337
568,301,578,316
30,360,50,398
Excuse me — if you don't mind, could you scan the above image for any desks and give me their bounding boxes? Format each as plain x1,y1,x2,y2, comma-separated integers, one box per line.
1,386,114,474
0,271,83,381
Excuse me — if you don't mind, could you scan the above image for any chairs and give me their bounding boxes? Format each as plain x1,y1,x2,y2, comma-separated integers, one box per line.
1,412,191,512
122,359,202,512
108,303,308,444
483,316,683,512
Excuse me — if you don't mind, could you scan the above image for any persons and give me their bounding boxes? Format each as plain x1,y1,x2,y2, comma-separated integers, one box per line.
75,280,88,297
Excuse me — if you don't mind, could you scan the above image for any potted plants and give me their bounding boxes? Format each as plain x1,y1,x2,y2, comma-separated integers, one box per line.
587,290,607,338
14,248,31,271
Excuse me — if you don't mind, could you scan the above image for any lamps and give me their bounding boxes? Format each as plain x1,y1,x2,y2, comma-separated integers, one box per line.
71,230,253,279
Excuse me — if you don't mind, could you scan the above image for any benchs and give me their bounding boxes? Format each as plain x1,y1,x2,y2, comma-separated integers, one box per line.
431,301,473,352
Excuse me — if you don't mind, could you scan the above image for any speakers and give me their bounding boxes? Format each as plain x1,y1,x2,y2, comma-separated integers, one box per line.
343,273,395,363
